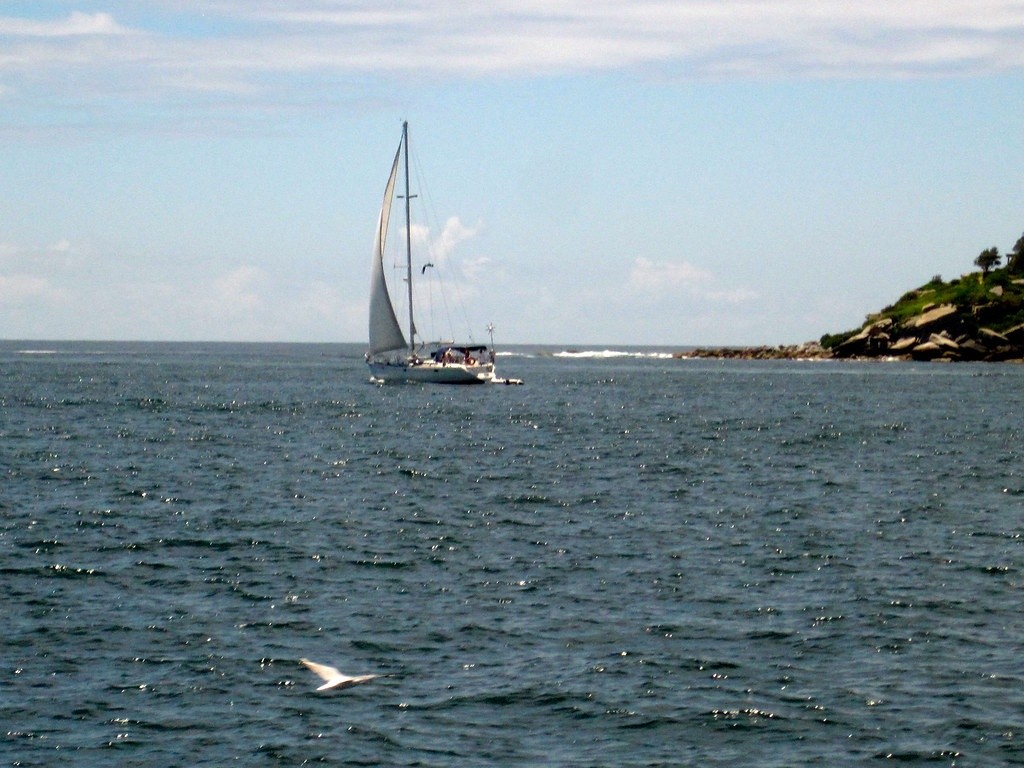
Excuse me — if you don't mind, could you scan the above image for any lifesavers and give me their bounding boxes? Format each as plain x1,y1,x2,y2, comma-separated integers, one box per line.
467,357,475,365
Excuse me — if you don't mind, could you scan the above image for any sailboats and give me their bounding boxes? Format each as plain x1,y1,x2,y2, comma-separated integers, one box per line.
360,119,498,386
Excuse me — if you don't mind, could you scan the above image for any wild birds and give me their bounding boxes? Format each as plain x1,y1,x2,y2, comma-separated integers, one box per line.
298,656,381,694
